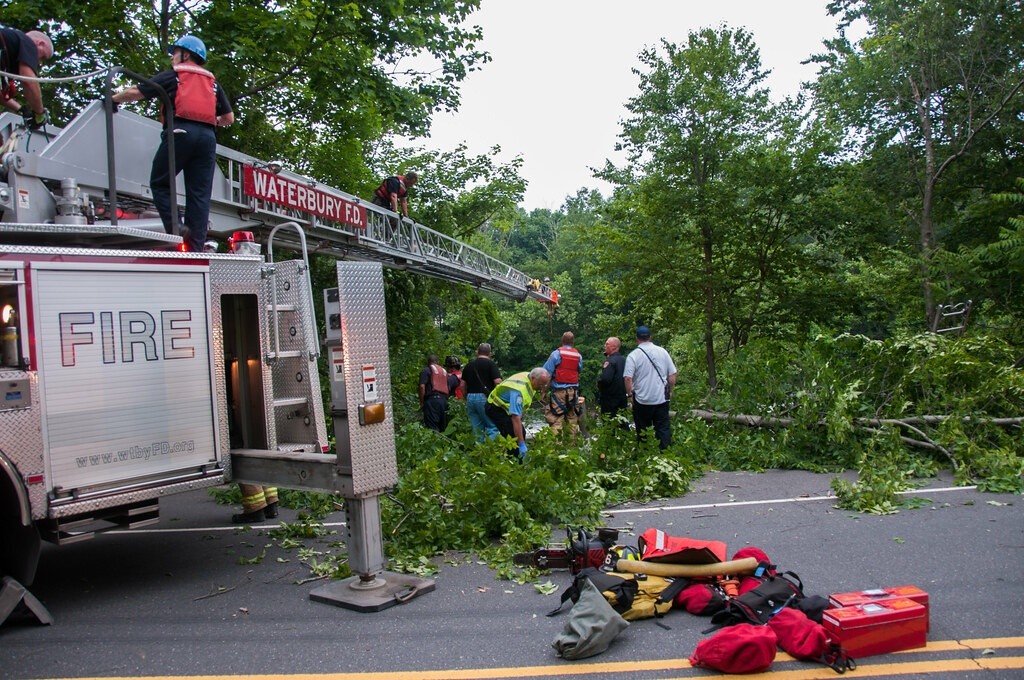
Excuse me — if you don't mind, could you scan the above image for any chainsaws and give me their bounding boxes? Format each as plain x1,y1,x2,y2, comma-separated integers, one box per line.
513,525,619,575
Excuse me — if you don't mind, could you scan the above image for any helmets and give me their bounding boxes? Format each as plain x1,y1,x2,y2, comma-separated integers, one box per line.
168,36,207,66
443,356,461,369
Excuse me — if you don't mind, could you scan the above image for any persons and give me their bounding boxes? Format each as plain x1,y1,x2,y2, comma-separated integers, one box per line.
623,326,678,453
597,336,630,431
484,367,551,465
418,354,449,433
0,26,54,133
371,171,417,242
445,356,466,400
543,331,583,446
102,36,234,252
459,343,503,443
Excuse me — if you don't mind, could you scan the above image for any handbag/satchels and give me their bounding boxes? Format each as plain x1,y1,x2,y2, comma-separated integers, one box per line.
544,528,858,674
664,384,671,401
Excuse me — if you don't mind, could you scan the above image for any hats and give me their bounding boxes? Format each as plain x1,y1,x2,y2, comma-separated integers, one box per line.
636,326,651,341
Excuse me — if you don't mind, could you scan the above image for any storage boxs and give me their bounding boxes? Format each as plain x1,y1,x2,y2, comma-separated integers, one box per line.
823,585,929,662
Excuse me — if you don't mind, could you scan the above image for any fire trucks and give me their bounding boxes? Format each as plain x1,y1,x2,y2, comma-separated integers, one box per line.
0,64,561,634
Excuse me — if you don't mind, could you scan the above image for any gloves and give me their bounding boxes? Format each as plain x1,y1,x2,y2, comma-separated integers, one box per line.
26,109,46,132
518,441,527,456
16,105,32,120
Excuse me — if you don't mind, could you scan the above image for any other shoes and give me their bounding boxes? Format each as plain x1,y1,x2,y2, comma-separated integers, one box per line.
178,221,192,242
264,502,279,519
232,508,266,523
